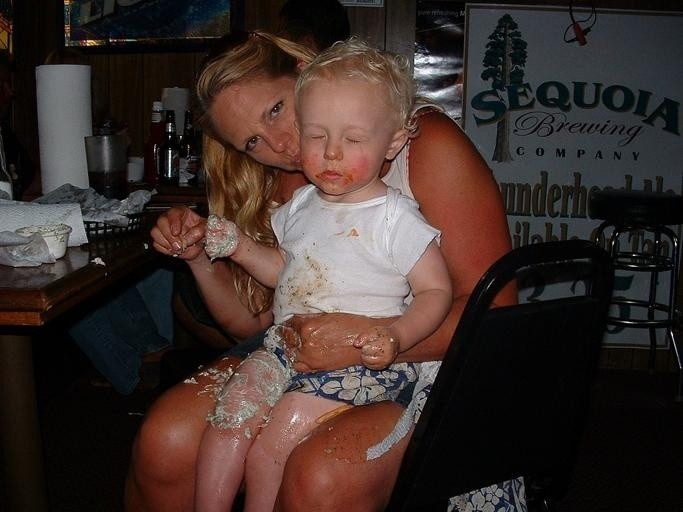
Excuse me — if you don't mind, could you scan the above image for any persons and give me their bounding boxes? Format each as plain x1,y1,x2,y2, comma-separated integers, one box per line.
0,47,35,201
194,39,453,512
279,0,351,53
121,31,519,512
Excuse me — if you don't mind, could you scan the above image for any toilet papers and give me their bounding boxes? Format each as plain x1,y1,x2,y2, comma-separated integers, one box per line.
34,63,92,195
162,87,190,136
0,199,89,247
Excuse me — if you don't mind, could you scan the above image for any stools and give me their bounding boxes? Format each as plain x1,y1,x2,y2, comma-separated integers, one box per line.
589,191,683,403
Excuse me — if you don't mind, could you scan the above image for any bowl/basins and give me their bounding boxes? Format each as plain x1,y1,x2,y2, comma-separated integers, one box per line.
15,224,72,260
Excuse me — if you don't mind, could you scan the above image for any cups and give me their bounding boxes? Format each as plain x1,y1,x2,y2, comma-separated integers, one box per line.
127,155,145,183
84,135,131,199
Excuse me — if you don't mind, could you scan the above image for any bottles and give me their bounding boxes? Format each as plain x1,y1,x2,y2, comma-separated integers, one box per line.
150,101,197,193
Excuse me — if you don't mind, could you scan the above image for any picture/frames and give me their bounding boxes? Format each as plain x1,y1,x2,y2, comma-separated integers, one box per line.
55,0,245,55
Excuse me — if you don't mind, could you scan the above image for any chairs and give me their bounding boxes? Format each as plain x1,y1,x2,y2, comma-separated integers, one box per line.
386,239,615,512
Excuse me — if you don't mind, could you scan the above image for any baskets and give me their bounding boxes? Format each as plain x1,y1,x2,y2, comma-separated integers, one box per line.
80,212,148,250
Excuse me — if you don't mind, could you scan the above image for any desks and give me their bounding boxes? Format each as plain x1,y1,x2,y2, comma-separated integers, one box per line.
0,181,207,512
